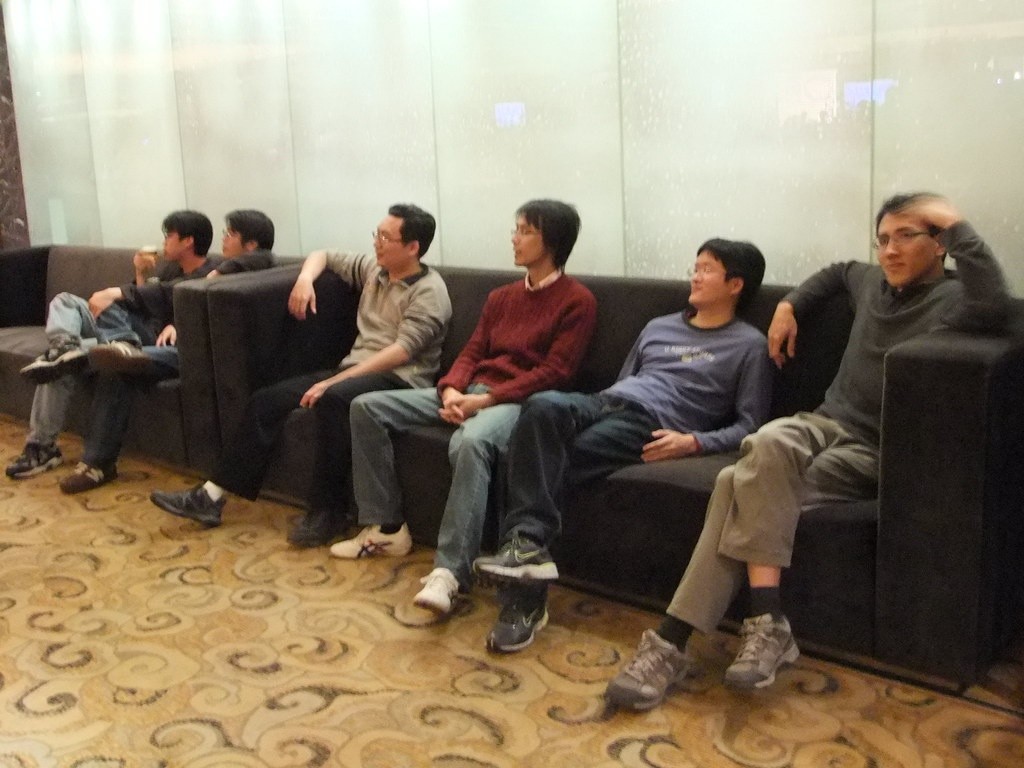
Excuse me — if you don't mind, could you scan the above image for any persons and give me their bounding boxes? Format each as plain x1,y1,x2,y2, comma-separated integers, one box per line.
328,199,596,619
606,191,1024,712
3,208,276,495
149,203,454,550
472,238,769,653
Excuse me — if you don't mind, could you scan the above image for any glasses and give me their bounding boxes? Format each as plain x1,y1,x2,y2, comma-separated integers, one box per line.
511,225,543,239
223,227,242,243
370,230,405,246
872,228,933,252
687,266,731,284
163,232,181,241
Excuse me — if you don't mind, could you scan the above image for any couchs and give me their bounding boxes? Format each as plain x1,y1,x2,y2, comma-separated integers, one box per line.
0,246,1024,675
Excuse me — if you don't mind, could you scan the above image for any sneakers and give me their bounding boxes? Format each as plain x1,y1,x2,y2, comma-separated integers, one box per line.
725,612,800,692
330,522,413,560
149,482,225,527
286,507,354,550
17,333,90,383
607,628,689,710
89,336,155,377
413,568,461,619
473,536,559,583
485,604,547,652
5,442,64,479
61,460,117,495
